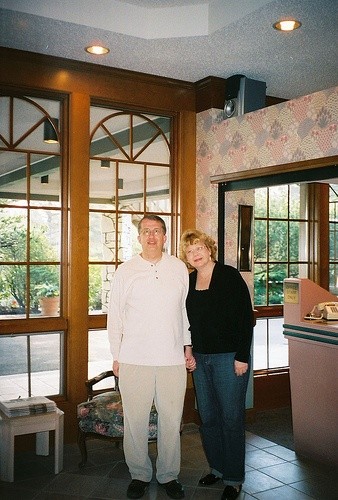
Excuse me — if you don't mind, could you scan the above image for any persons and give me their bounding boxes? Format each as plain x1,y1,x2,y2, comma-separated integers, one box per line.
107,213,196,499
181,228,254,500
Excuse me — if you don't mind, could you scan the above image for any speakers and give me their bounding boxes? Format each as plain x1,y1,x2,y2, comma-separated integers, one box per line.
223,77,266,119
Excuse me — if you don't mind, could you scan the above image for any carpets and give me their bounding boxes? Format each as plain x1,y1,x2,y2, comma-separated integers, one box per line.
246,407,295,451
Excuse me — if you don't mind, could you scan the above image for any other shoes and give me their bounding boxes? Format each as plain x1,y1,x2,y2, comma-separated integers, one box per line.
221,483,242,500
199,473,224,487
127,479,150,499
157,477,184,499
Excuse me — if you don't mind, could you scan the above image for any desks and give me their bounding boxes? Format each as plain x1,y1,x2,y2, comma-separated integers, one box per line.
0,408,65,483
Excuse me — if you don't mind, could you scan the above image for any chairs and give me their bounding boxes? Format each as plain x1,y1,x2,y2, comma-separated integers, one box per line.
77,371,185,472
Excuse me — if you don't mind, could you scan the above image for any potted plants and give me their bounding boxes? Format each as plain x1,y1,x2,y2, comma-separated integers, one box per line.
35,281,60,315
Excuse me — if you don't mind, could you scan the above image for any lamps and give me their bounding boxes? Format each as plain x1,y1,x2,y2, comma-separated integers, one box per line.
41,175,48,184
43,118,59,143
118,179,123,189
101,161,110,168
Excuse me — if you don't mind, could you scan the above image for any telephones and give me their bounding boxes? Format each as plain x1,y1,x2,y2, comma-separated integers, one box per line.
316,300,338,321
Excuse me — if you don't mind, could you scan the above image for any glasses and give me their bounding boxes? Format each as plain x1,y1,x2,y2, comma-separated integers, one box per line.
140,228,163,237
